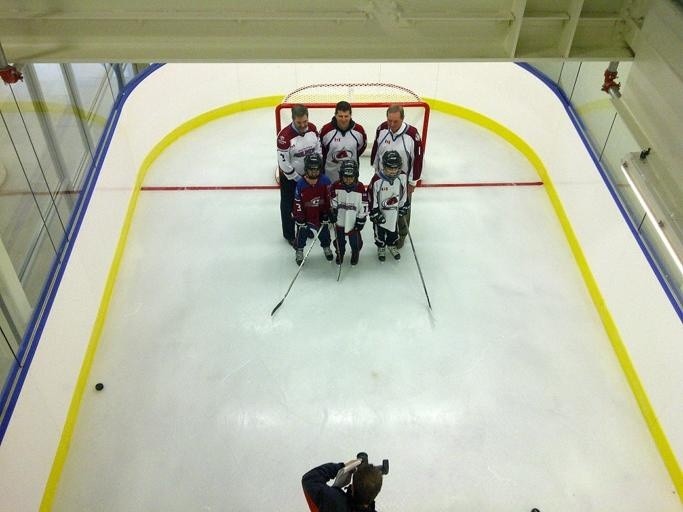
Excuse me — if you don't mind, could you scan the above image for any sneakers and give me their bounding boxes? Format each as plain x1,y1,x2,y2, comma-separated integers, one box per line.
296,249,304,260
336,251,344,265
307,230,314,238
288,238,296,245
387,246,400,260
323,247,334,261
350,251,359,265
378,247,386,260
396,232,407,248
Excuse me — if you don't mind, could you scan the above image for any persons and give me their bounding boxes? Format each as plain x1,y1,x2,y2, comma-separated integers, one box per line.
274,105,324,249
364,150,411,262
318,99,367,188
368,104,423,251
299,457,383,511
289,153,335,267
322,158,369,267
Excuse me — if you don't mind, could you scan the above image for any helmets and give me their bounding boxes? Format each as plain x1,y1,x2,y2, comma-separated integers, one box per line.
304,152,322,179
338,159,359,189
381,150,401,182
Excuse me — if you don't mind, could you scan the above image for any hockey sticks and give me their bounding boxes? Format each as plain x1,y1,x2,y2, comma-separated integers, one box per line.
327,185,341,281
404,215,439,321
271,224,323,315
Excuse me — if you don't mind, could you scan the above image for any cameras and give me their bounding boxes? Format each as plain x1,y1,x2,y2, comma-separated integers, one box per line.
355,453,389,475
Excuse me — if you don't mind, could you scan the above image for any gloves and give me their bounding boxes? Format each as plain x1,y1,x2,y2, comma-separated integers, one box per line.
398,206,407,217
369,210,386,223
330,209,338,223
354,218,366,231
297,222,308,232
320,216,331,225
